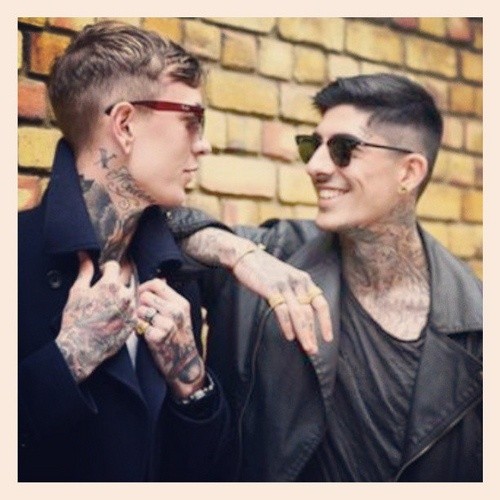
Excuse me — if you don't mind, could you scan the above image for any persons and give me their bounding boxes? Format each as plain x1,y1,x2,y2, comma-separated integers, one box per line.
18,19,253,484
164,72,481,482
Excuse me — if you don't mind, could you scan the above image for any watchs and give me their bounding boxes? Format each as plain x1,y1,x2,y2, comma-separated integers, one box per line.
171,372,215,411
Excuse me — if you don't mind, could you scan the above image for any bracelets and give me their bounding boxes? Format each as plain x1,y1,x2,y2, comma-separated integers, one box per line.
231,242,266,271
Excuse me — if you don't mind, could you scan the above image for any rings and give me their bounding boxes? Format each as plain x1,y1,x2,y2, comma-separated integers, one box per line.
270,297,284,308
136,322,150,334
298,294,310,303
145,307,158,322
309,287,323,298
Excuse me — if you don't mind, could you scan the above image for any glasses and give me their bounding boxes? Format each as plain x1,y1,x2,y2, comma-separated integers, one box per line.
104,100,204,134
295,135,413,168
